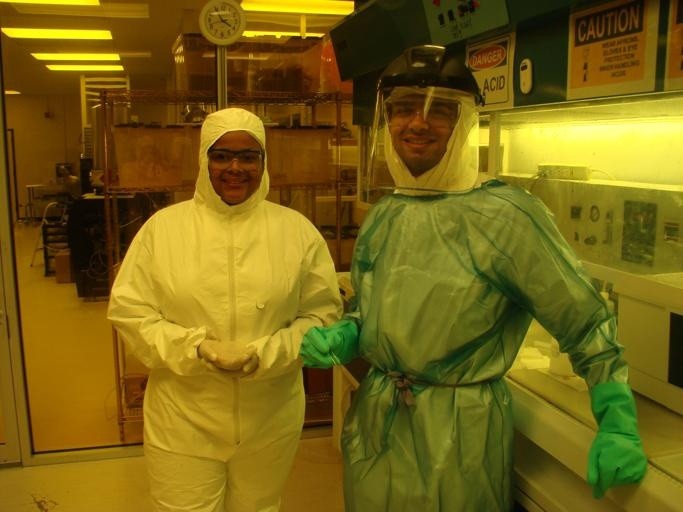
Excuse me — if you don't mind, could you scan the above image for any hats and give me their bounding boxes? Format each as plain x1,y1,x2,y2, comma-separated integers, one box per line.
363,42,481,197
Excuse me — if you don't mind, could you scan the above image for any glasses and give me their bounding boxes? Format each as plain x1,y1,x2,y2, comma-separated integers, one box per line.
208,149,262,173
382,99,457,129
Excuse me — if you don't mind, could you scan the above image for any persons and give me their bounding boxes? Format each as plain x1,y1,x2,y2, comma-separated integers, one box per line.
105,106,344,510
297,42,651,510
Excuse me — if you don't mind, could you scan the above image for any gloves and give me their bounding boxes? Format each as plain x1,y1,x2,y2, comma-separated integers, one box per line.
297,319,359,369
585,381,648,501
198,341,258,381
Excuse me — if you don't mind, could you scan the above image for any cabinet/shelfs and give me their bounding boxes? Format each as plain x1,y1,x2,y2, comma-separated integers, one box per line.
334,116,682,511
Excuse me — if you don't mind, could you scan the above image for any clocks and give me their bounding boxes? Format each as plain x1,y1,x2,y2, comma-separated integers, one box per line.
197,0,247,46
